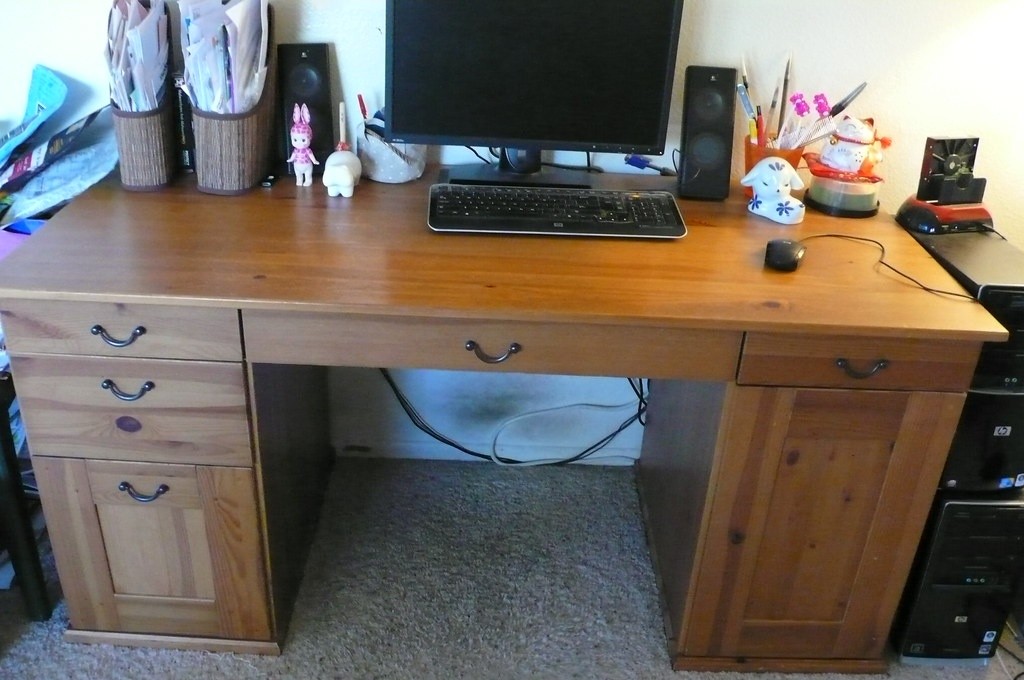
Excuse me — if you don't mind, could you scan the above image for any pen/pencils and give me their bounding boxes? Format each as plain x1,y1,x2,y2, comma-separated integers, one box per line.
357,94,369,120
735,55,869,169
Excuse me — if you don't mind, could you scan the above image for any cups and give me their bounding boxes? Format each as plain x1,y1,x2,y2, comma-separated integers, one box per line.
745,133,805,198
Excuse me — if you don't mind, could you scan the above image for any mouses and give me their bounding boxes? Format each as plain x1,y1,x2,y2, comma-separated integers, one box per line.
765,239,807,272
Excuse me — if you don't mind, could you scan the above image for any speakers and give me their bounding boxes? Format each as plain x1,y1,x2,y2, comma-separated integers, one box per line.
677,66,738,200
275,43,335,176
905,228,1024,498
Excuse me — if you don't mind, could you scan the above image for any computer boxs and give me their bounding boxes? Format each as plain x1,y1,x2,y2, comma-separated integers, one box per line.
891,488,1024,664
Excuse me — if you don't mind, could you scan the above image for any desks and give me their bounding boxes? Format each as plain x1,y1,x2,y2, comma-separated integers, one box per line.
0,167,1009,674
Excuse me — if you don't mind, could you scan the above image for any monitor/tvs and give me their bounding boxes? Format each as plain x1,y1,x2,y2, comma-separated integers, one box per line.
384,0,684,188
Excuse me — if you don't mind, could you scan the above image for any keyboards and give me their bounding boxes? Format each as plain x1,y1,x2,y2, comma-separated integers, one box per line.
427,184,688,238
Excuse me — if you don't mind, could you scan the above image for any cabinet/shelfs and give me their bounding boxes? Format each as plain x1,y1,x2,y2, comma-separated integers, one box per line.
0,299,332,657
635,334,987,676
